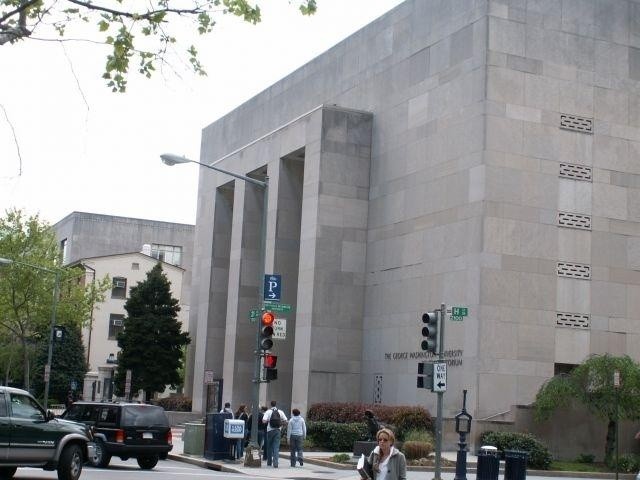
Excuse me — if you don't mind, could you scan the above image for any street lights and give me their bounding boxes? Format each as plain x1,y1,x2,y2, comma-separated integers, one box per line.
0,258,59,411
160,149,269,447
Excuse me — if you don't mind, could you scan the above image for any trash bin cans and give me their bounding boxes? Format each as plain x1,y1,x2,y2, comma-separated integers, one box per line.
475,450,529,480
184,423,205,455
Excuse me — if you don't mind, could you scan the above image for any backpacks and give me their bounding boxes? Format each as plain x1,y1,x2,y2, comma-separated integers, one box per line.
269,407,282,428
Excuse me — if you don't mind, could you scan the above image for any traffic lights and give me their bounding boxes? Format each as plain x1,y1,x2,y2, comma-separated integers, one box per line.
417,363,433,389
421,312,437,351
260,313,277,381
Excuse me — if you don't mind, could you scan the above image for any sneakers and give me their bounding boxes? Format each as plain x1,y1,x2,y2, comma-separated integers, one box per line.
261,456,305,469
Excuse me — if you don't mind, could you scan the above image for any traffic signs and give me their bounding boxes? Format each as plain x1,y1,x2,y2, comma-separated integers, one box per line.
434,364,447,392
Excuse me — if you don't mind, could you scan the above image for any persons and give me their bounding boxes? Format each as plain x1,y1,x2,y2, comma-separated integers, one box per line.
219,400,269,463
286,409,307,467
261,400,288,468
359,428,408,480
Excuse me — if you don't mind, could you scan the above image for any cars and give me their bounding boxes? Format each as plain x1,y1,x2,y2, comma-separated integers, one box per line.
0,386,173,480
